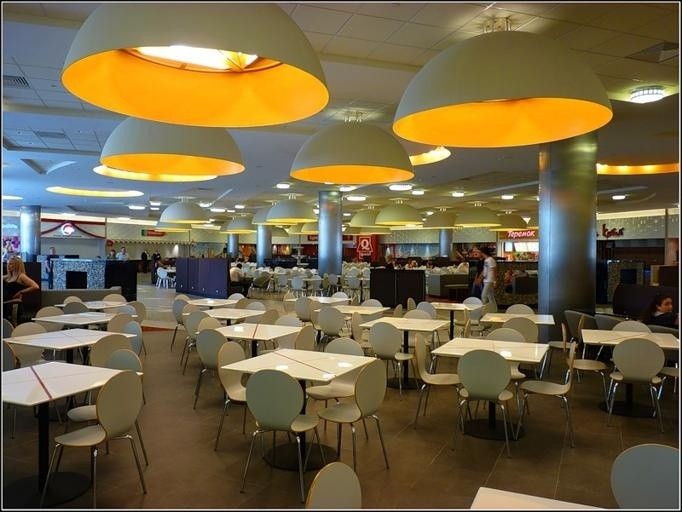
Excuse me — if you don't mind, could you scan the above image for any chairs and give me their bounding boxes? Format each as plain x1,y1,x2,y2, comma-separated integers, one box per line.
451,350,517,457
606,337,665,433
305,462,362,510
40,370,148,508
214,341,247,452
239,369,327,504
303,359,390,474
414,333,465,432
520,341,576,446
170,261,678,410
609,445,678,508
4,264,150,473
152,262,177,290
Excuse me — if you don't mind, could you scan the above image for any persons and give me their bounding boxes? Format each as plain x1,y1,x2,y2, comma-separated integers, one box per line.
229,263,251,296
3,256,40,318
45,247,59,289
105,247,164,284
643,295,679,328
474,247,497,303
385,254,436,268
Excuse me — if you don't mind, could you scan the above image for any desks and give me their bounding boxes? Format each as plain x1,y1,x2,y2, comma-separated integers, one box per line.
471,487,596,508
5,362,128,496
221,348,377,470
581,329,677,418
431,338,549,441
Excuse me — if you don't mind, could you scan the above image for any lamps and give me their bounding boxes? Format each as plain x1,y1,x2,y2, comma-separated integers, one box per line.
360,105,452,167
321,185,541,237
99,116,245,179
392,19,614,148
288,111,415,185
630,87,665,104
46,162,320,236
614,195,626,202
61,5,329,128
